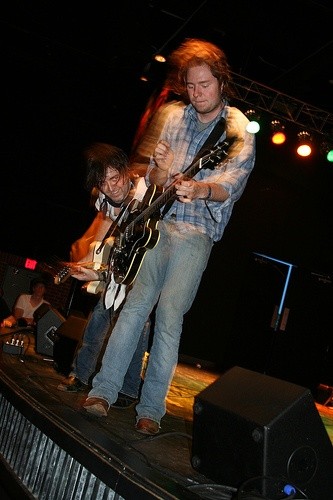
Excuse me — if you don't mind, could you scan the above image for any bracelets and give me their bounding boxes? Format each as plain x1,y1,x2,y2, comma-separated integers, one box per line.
197,184,211,200
92,270,107,283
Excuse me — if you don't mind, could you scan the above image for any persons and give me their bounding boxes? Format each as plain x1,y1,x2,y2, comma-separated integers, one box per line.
13,281,51,329
56,142,149,410
0,295,17,327
78,40,255,435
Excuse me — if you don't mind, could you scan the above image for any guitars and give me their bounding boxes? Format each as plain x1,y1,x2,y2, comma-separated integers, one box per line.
41,241,126,311
111,132,244,286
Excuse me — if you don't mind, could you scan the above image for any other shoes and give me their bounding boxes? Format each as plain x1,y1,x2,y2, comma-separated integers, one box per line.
136,419,160,435
83,397,108,417
57,376,89,393
111,392,139,409
